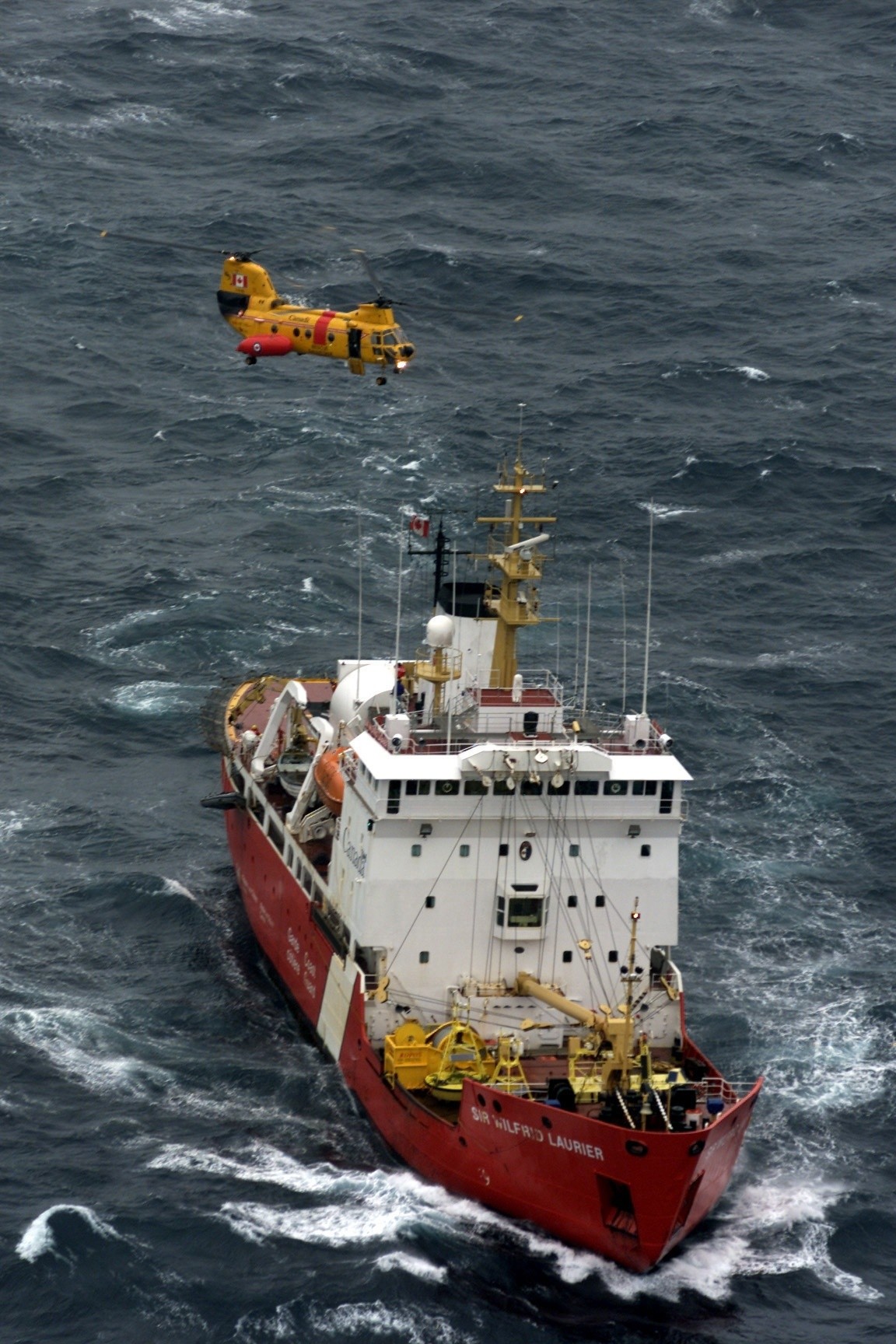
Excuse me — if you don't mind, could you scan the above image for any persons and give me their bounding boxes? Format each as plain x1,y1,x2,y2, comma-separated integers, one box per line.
657,1089,668,1111
594,1031,612,1062
390,662,409,696
353,330,388,357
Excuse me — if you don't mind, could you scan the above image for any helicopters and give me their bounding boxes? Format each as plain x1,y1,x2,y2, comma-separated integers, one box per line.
100,228,415,386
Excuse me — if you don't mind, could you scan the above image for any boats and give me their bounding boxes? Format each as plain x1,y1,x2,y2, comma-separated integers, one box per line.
206,398,769,1275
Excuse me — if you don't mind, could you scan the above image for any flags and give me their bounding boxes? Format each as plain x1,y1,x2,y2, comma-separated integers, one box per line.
410,515,430,537
